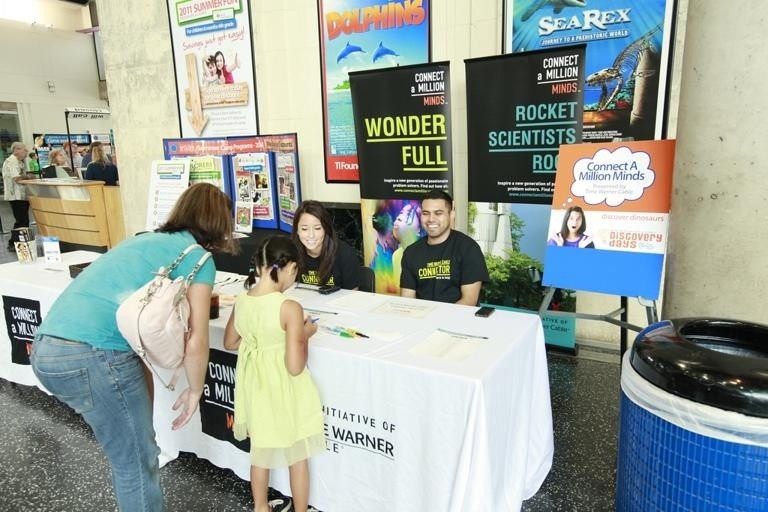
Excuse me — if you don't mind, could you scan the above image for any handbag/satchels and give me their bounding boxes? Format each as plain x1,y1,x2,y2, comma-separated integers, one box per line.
114,243,214,392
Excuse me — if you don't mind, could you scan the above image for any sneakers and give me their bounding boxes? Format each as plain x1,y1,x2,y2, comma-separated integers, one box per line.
268,496,292,511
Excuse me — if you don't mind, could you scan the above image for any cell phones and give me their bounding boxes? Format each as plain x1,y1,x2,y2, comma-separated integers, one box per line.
474,306,495,318
319,285,340,294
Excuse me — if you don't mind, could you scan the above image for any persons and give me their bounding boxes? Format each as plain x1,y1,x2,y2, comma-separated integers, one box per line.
398,188,491,306
28,180,239,512
205,51,241,85
546,206,595,250
206,55,226,85
290,199,361,292
24,135,118,188
222,236,327,512
2,141,37,253
391,200,421,281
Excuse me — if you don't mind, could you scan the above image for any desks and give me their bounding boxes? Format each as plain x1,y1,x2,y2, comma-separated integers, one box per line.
1,249,558,511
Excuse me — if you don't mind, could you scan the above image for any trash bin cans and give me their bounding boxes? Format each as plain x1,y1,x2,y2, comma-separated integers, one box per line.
615,316,767,512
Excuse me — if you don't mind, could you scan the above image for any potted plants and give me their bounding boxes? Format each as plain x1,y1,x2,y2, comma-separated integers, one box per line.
473,248,579,355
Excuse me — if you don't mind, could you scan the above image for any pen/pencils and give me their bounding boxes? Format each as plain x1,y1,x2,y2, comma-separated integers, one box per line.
304,319,319,323
320,324,370,338
